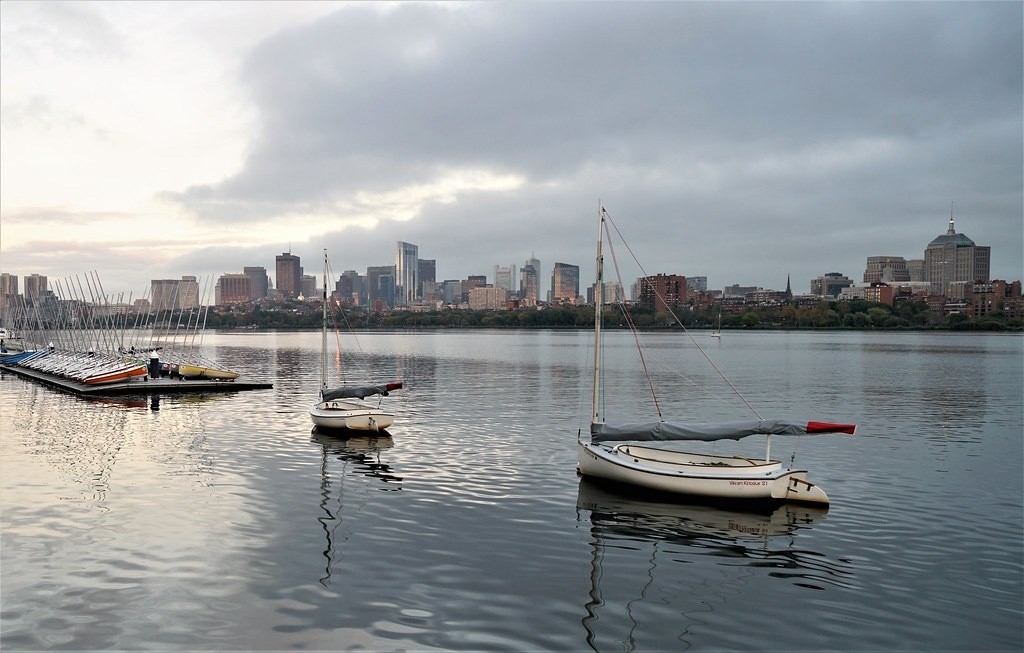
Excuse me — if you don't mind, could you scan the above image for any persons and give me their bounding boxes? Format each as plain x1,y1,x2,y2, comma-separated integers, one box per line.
1,339,5,353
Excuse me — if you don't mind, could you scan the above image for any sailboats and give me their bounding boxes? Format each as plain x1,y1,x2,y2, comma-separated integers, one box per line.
311,424,393,584
0,269,240,385
574,199,829,506
574,474,825,653
315,245,398,430
711,312,722,337
